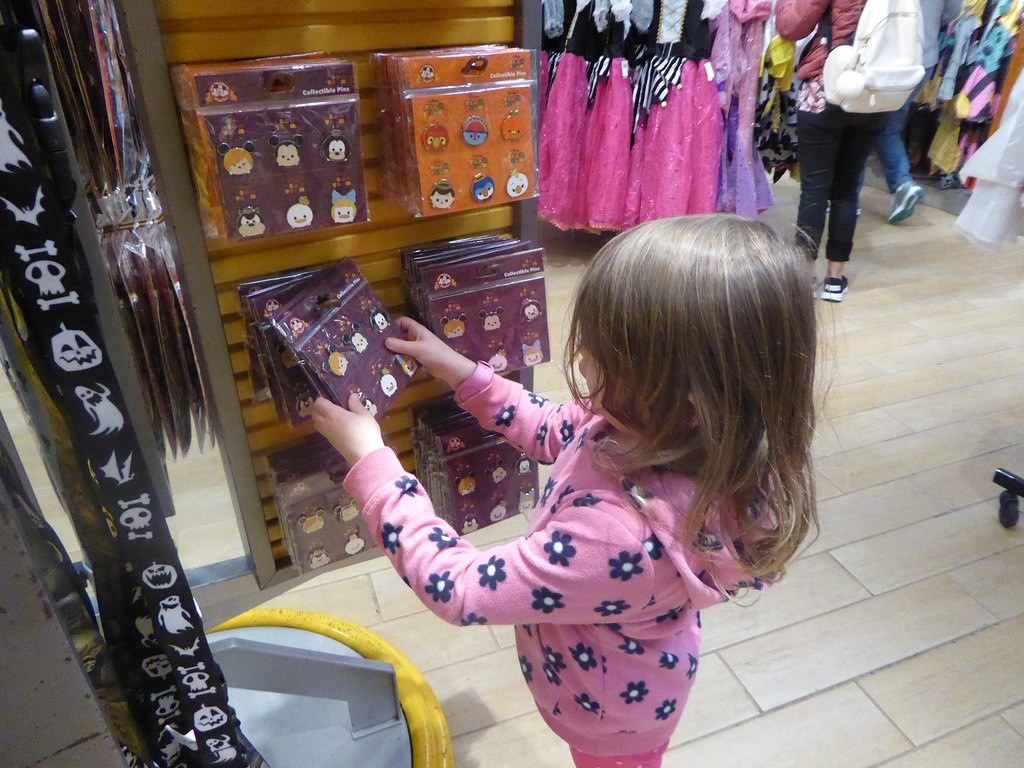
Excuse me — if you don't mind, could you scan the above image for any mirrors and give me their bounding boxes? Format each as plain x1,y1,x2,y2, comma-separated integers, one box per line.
0,361,255,591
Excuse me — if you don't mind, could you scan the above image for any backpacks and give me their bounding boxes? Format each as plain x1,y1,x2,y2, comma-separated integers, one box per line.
824,0,925,113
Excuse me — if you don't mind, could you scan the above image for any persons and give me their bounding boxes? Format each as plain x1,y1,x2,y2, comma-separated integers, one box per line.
854,0,962,225
774,0,891,304
310,210,836,768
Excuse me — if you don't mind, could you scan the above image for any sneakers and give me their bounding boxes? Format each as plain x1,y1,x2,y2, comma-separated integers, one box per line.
821,274,850,301
887,181,925,221
